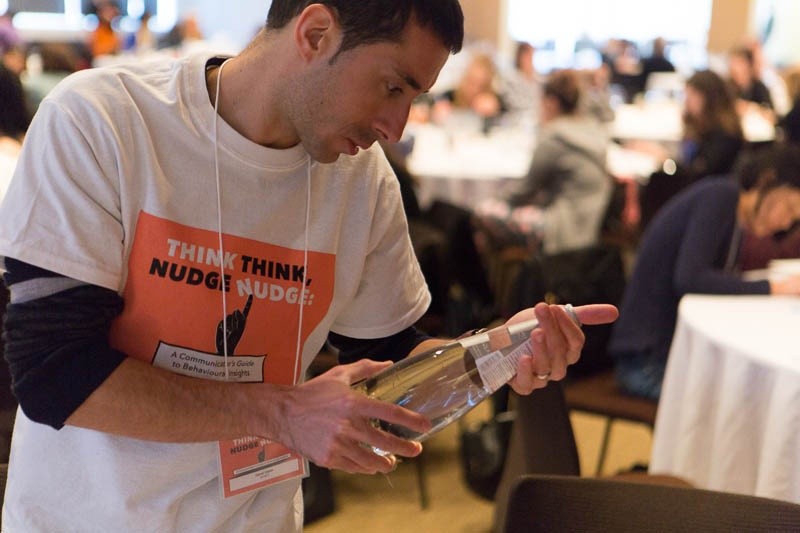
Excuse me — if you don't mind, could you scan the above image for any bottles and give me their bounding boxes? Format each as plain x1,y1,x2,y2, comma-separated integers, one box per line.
348,304,581,457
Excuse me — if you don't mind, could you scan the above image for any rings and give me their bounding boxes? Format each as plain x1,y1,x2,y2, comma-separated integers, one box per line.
323,35,800,402
533,371,551,380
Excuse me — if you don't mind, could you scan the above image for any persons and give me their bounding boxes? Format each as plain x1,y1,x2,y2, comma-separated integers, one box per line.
0,0,200,208
0,0,618,533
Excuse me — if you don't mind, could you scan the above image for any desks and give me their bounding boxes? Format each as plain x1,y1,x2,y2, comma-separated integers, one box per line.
401,72,777,253
646,268,800,506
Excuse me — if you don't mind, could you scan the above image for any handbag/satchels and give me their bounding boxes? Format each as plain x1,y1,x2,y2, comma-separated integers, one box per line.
461,416,530,504
600,180,629,236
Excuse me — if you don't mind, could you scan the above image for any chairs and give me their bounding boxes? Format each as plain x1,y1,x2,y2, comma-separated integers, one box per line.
478,246,800,533
